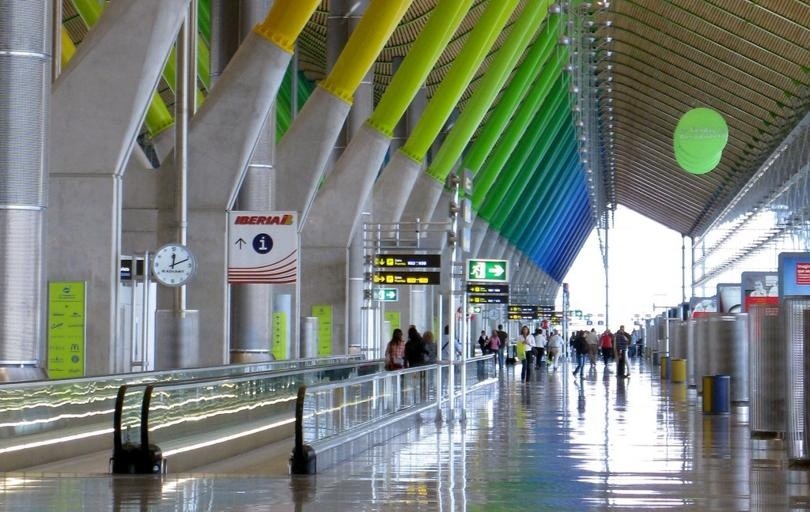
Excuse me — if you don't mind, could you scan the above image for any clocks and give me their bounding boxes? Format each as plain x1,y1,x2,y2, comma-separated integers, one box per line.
149,241,198,288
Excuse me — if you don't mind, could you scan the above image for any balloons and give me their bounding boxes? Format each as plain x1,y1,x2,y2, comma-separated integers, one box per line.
673,108,729,176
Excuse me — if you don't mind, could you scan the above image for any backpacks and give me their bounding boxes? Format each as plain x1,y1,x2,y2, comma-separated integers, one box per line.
531,345,539,356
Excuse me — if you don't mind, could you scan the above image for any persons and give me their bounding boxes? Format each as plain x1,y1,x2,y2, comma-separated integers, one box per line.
443,324,462,360
385,329,406,370
403,324,431,366
479,325,642,381
423,331,437,362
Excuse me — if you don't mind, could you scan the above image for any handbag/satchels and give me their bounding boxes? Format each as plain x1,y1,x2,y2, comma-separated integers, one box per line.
384,358,403,371
516,341,528,362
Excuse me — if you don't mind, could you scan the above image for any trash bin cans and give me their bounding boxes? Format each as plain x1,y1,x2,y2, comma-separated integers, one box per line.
702,375,730,414
671,359,688,383
660,357,673,379
